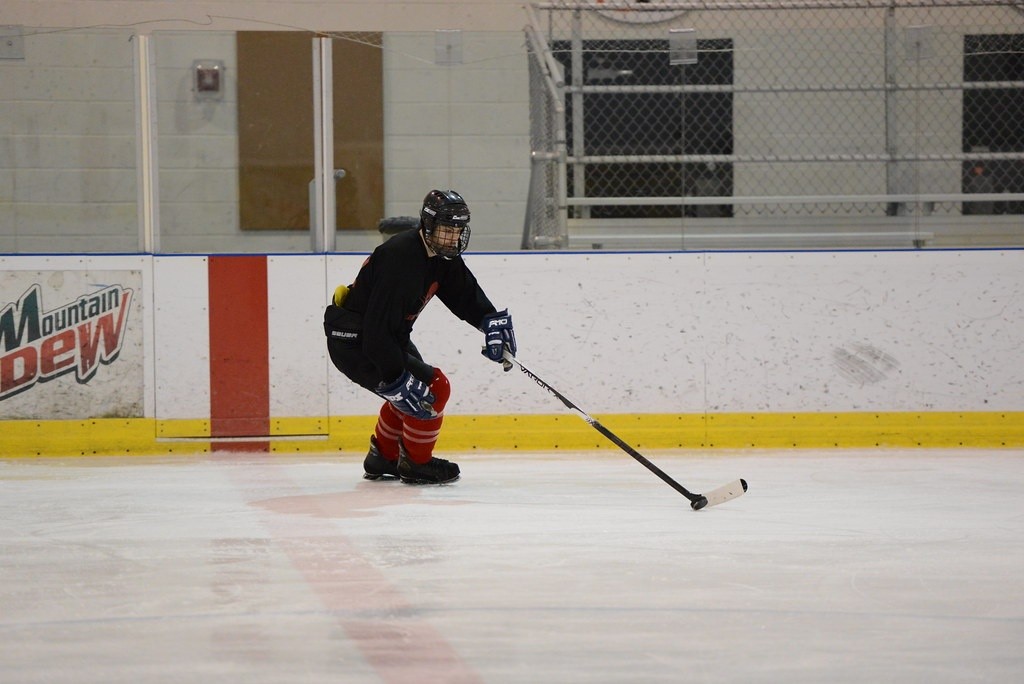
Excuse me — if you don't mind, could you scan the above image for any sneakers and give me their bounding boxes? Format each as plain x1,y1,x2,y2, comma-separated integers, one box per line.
399,442,459,484
364,435,398,480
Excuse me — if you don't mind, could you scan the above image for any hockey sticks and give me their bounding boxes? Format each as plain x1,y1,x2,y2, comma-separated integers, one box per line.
502,347,751,512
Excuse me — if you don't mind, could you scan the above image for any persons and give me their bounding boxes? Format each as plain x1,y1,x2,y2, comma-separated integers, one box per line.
324,190,515,484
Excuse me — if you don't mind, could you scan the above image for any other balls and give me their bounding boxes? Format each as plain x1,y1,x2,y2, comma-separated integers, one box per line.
690,496,708,510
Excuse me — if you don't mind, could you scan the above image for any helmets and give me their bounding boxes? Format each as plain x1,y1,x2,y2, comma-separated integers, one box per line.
420,189,471,260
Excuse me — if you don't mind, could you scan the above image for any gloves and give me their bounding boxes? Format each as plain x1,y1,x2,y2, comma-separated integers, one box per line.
481,308,516,372
375,371,437,419
